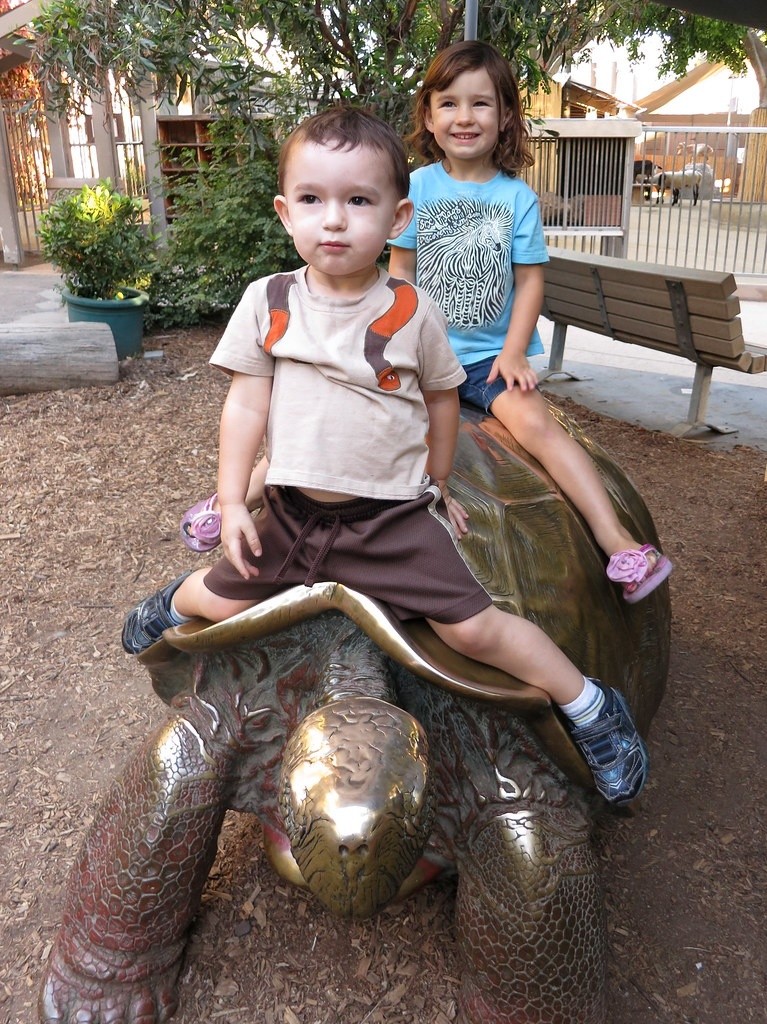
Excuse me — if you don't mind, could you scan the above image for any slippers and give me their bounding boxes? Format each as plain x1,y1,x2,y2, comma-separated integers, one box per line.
179,493,222,552
606,543,671,603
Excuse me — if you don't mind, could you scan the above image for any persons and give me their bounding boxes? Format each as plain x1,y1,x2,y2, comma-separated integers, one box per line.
116,109,652,804
180,42,673,606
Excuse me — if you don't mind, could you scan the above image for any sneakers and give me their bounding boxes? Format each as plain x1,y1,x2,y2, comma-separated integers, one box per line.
121,571,195,654
569,676,648,802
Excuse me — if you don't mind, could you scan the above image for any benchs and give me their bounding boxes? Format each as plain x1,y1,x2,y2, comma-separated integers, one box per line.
542,246,767,441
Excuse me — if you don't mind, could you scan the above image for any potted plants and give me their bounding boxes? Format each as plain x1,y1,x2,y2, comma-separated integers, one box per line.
35,183,152,363
